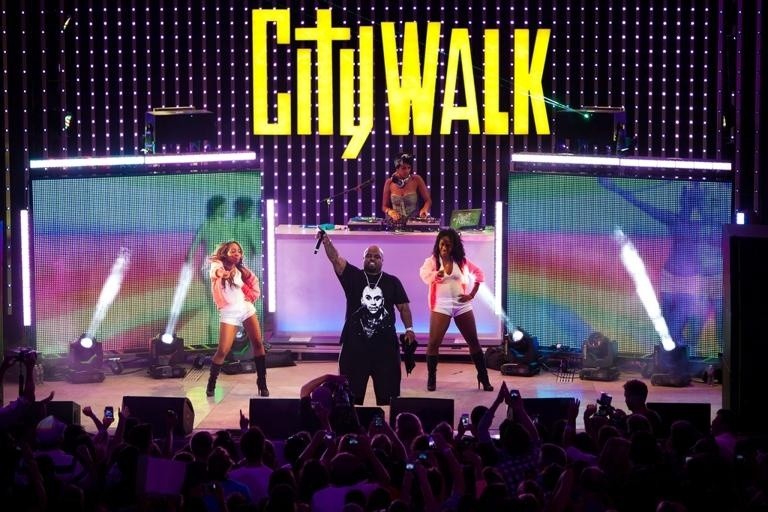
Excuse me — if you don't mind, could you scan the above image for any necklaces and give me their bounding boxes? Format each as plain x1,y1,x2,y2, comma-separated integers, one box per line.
444,256,451,267
364,270,384,289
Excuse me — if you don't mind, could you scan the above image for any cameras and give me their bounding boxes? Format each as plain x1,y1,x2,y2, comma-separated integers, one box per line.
406,463,416,470
595,392,614,414
419,453,426,459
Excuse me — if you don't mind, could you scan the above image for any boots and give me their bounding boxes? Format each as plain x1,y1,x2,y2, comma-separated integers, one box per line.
206,363,224,396
254,355,269,396
471,352,493,391
426,355,438,391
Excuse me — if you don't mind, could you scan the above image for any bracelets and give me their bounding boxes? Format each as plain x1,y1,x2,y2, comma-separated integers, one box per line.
404,327,414,333
324,239,332,247
468,293,475,301
385,206,391,216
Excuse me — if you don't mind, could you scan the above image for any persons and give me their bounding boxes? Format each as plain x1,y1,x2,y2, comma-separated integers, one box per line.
685,183,727,358
382,154,434,221
311,226,417,409
595,175,707,348
2,347,767,510
418,228,495,393
207,240,270,398
222,197,264,327
184,194,239,348
351,283,392,341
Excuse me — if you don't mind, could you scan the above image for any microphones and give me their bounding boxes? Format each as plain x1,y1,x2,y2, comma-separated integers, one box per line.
359,177,375,190
313,230,325,254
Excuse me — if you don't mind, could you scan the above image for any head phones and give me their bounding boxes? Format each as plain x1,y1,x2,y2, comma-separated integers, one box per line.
390,172,411,188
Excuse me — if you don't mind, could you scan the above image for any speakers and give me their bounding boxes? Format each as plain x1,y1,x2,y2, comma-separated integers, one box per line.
355,407,385,426
144,106,215,155
554,106,630,157
389,397,454,433
645,403,710,431
120,396,194,435
249,399,302,441
507,398,576,436
10,401,81,427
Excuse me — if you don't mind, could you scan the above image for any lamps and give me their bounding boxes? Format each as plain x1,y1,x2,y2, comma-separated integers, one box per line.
500,325,691,390
64,332,187,385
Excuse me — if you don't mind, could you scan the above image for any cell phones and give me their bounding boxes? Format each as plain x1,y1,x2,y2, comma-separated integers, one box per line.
105,406,113,419
428,436,435,448
461,413,469,430
